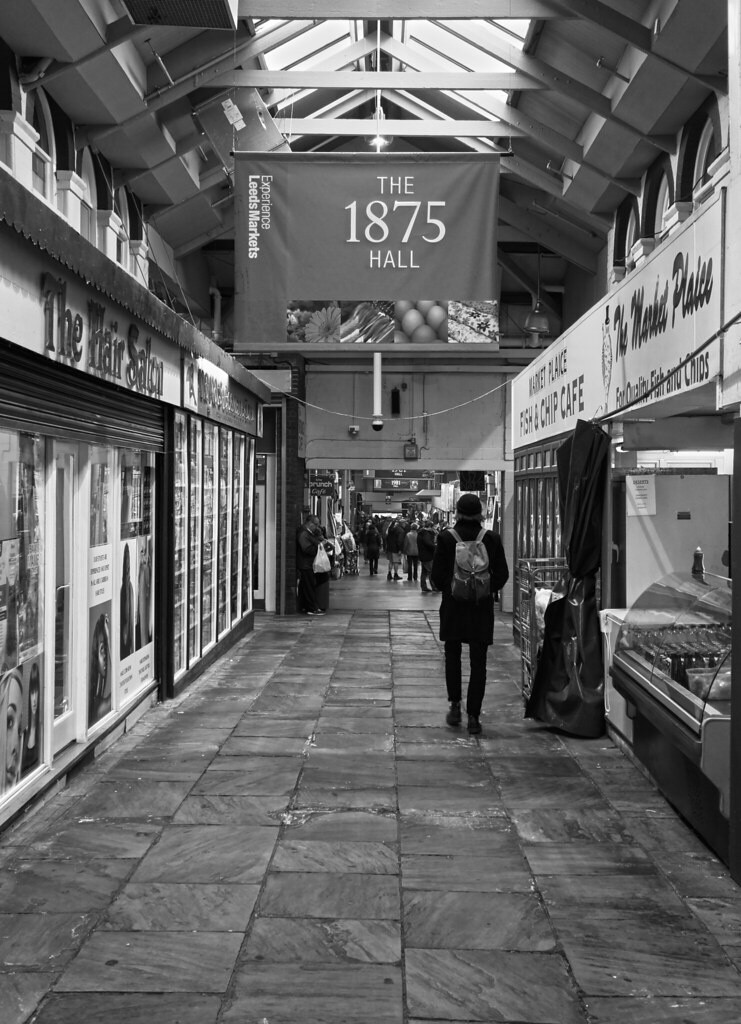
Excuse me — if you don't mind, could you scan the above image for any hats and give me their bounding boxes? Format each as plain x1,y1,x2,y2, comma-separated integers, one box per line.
457,494,482,517
399,518,407,523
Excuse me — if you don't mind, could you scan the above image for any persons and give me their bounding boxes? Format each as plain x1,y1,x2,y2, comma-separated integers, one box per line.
431,493,510,732
0,663,40,796
355,510,448,592
296,514,334,615
136,542,151,648
120,543,134,662
88,614,111,728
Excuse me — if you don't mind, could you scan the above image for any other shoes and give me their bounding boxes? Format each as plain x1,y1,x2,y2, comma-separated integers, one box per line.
386,574,393,581
373,568,378,574
446,711,461,724
307,608,327,616
408,578,412,580
422,588,431,591
468,722,481,734
414,577,417,580
394,573,402,580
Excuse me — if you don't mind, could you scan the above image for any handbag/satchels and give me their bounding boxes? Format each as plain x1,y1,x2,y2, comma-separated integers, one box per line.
400,554,408,573
324,541,335,568
313,542,331,574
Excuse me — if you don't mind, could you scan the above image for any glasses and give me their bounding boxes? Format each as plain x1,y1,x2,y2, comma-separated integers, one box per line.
311,521,319,527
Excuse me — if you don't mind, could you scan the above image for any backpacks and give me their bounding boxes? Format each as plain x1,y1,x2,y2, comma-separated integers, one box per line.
446,528,491,600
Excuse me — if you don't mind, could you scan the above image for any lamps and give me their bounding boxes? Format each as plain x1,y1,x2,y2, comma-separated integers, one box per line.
392,480,401,488
522,245,551,336
392,469,406,478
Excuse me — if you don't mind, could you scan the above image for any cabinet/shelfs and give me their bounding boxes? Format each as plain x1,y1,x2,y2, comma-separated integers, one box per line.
608,567,732,868
517,557,569,706
